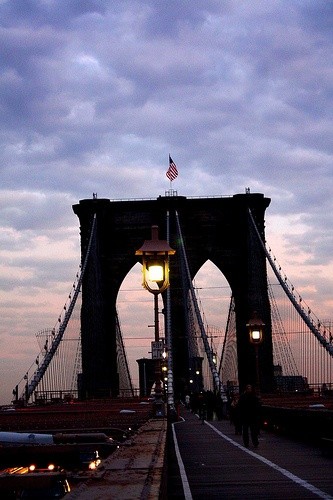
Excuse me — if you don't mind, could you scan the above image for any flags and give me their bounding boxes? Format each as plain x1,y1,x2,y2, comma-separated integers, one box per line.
166,156,179,182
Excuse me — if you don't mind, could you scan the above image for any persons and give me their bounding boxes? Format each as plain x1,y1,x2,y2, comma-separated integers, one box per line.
237,384,263,448
184,388,242,437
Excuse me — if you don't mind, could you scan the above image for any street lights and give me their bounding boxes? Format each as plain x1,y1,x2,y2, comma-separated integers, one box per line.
243,307,267,396
134,223,177,340
